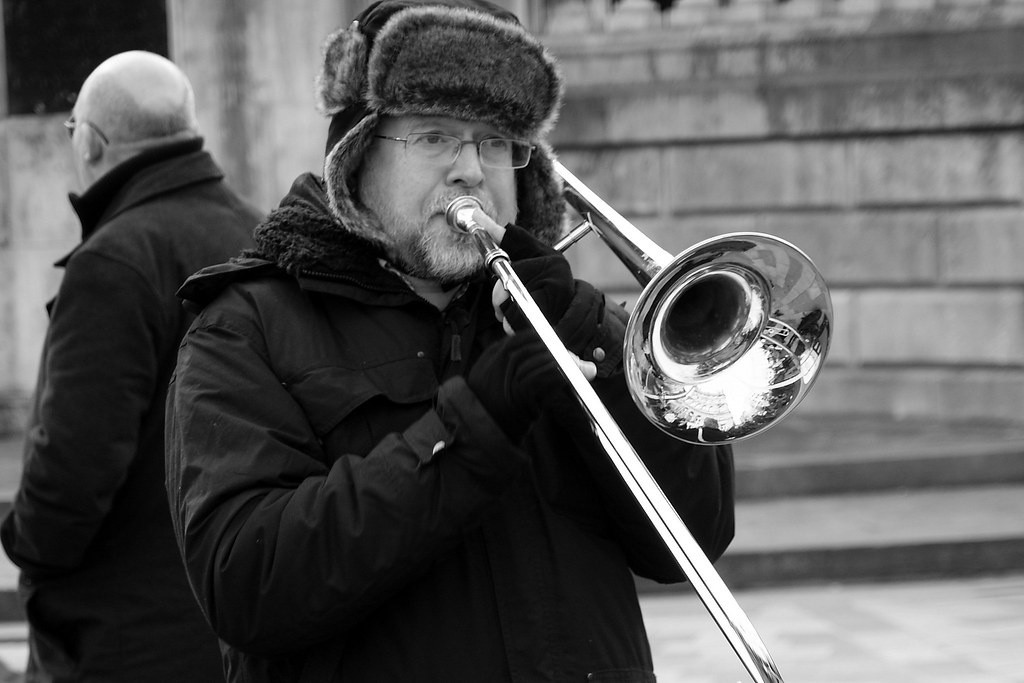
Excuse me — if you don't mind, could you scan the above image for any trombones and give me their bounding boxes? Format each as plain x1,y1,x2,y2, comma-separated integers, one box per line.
443,151,835,682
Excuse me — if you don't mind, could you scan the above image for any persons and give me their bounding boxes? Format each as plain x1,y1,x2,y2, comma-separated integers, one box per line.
162,0,737,683
0,50,264,683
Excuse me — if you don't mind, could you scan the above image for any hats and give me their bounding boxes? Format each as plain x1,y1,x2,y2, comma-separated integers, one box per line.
316,0,569,255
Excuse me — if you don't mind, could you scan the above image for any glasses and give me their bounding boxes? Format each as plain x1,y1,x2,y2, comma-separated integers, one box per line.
370,131,536,170
61,116,110,151
